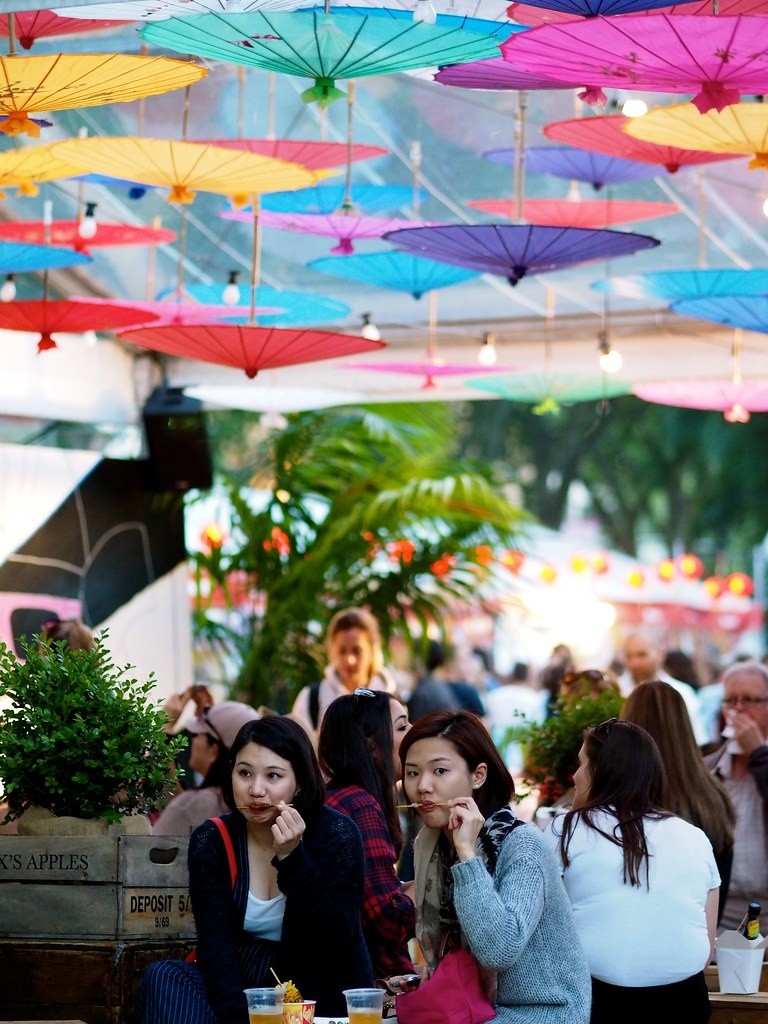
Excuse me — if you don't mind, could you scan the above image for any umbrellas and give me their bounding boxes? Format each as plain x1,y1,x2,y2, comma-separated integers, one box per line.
0,0,768,424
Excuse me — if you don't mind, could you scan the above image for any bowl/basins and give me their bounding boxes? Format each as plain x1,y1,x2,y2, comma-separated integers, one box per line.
280,1000,316,1024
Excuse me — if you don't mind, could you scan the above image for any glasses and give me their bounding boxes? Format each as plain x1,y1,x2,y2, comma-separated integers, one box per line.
595,717,618,736
353,686,376,702
722,694,768,710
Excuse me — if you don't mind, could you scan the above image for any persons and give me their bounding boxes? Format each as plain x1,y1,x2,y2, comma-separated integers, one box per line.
543,717,721,1024
398,708,594,1024
125,716,375,1024
40,608,768,980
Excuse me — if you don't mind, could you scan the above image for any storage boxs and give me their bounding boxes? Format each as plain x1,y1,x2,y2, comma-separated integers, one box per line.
0,835,198,940
715,930,768,994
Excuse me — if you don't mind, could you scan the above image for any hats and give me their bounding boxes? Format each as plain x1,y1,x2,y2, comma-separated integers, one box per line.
188,700,259,748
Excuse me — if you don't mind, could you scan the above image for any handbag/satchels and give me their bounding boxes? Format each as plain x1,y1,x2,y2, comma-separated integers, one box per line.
395,931,496,1024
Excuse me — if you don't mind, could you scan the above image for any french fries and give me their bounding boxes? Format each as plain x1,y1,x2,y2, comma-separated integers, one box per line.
282,1004,316,1024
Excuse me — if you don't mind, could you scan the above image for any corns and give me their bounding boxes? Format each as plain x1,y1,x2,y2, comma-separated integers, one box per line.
275,980,305,1004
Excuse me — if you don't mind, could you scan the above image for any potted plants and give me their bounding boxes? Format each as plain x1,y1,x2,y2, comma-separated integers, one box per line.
1,629,190,834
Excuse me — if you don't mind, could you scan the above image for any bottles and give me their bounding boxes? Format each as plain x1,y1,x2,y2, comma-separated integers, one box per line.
742,901,762,940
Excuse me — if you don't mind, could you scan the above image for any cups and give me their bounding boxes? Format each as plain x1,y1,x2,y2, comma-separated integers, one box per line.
341,988,387,1024
243,987,284,1024
